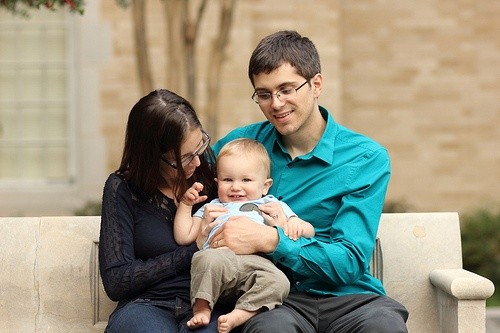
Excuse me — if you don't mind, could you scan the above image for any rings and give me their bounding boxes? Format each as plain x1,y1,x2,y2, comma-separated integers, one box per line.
272,215,278,218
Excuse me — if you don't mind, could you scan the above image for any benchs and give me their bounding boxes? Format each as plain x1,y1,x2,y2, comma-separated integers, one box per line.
1,212,494,333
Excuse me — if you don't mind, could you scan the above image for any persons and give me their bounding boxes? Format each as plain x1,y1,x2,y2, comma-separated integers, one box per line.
173,138,314,333
98,88,288,333
208,30,409,333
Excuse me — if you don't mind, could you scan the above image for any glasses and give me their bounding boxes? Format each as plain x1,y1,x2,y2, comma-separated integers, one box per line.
251,79,311,104
159,130,211,170
